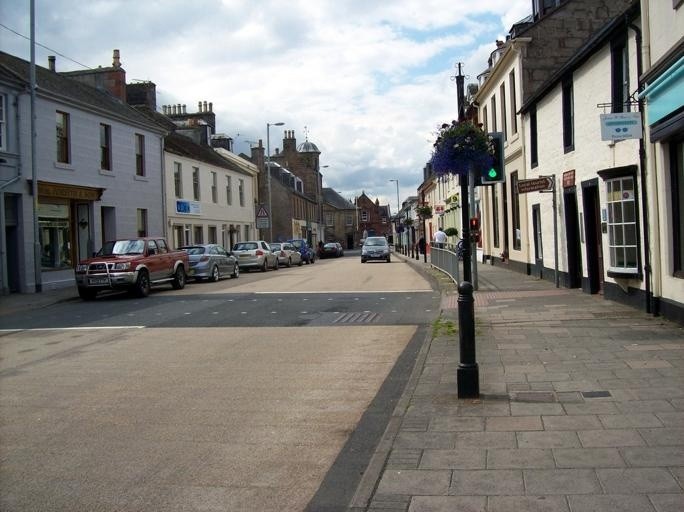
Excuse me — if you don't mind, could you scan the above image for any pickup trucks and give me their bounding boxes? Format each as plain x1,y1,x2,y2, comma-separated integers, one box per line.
75,237,189,302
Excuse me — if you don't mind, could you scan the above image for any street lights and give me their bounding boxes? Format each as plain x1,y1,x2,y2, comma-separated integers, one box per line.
317,165,329,241
389,180,401,252
445,59,479,399
267,122,286,242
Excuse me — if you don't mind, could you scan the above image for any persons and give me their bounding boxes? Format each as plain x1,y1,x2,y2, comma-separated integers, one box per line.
432,228,448,248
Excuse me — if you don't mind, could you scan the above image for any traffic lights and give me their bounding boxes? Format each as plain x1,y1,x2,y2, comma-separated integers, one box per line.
470,218,481,243
481,132,504,184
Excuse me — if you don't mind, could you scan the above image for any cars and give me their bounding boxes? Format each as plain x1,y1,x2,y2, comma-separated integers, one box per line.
176,239,316,282
319,242,344,258
359,236,390,263
